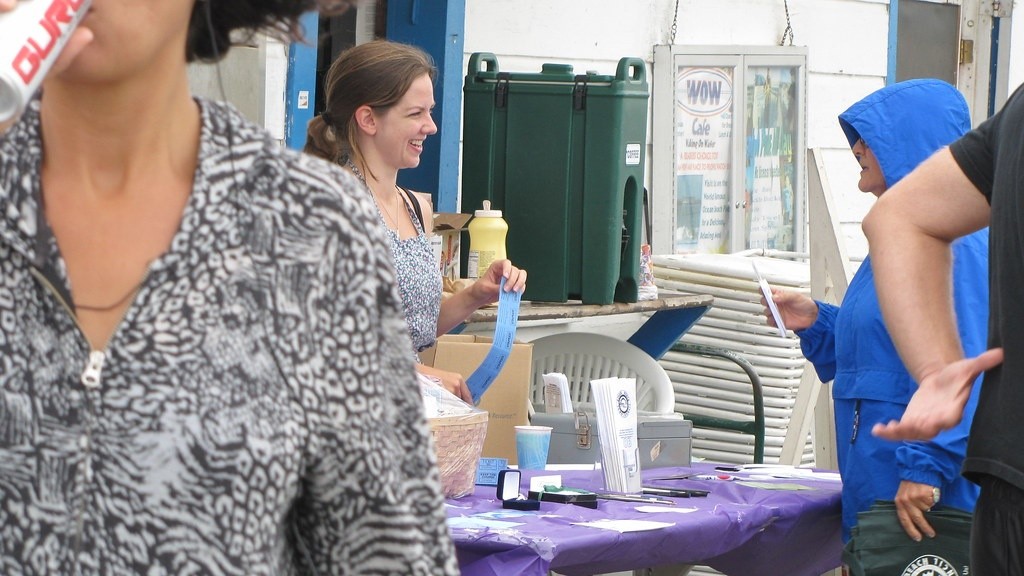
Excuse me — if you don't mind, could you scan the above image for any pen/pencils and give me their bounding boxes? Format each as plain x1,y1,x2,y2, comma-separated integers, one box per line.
715,466,738,471
643,490,692,498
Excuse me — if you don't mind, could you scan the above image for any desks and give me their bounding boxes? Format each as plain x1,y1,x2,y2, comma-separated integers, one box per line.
441,464,842,575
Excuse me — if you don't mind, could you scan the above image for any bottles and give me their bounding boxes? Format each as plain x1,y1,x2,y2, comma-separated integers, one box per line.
466,200,508,283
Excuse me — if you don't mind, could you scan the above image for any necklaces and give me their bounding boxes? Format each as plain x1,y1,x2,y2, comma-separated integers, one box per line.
370,184,399,237
72,284,144,313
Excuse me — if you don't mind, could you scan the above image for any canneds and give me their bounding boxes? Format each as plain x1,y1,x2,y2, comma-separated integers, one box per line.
1,0,92,129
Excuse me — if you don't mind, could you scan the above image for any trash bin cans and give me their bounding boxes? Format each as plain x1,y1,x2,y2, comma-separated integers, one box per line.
464,50,655,306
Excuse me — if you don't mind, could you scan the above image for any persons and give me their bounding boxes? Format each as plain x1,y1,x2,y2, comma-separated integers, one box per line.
759,80,991,574
1,0,458,575
857,83,1024,576
301,37,530,406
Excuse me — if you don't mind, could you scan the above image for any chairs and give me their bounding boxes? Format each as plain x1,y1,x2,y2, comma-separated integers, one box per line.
529,332,675,414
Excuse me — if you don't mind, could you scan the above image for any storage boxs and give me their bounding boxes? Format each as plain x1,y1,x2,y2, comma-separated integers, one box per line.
419,333,535,465
433,210,473,280
530,411,695,468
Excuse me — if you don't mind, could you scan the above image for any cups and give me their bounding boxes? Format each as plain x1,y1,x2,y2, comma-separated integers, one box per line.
513,425,554,472
